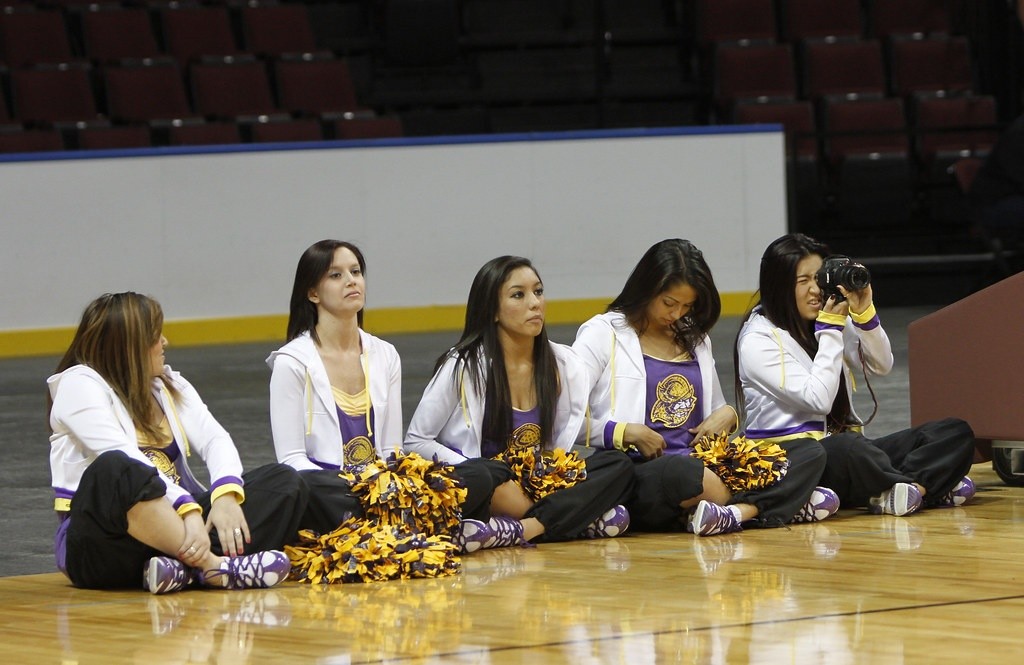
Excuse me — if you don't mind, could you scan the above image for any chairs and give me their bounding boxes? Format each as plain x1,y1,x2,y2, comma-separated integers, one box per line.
692,1,998,231
0,0,403,153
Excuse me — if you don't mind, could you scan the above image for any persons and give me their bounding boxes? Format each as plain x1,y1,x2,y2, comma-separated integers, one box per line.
733,233,976,517
47,292,309,593
973,112,1024,235
571,238,839,536
265,239,404,543
403,254,634,554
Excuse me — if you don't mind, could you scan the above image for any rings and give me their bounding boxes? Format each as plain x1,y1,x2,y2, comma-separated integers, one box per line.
234,528,240,535
844,292,851,298
190,546,196,553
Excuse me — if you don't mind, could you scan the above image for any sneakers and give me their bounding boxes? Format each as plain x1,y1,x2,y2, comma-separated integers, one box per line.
150,596,190,635
866,483,924,517
197,549,291,589
580,540,633,573
693,534,739,578
443,518,492,556
479,515,526,549
576,504,631,540
214,589,293,628
878,516,924,552
929,476,976,508
945,510,976,538
756,486,840,524
686,499,745,536
460,574,490,591
485,549,525,580
145,556,201,595
784,524,841,560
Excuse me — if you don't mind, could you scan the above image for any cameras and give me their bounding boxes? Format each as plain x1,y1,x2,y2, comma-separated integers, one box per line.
817,257,871,306
